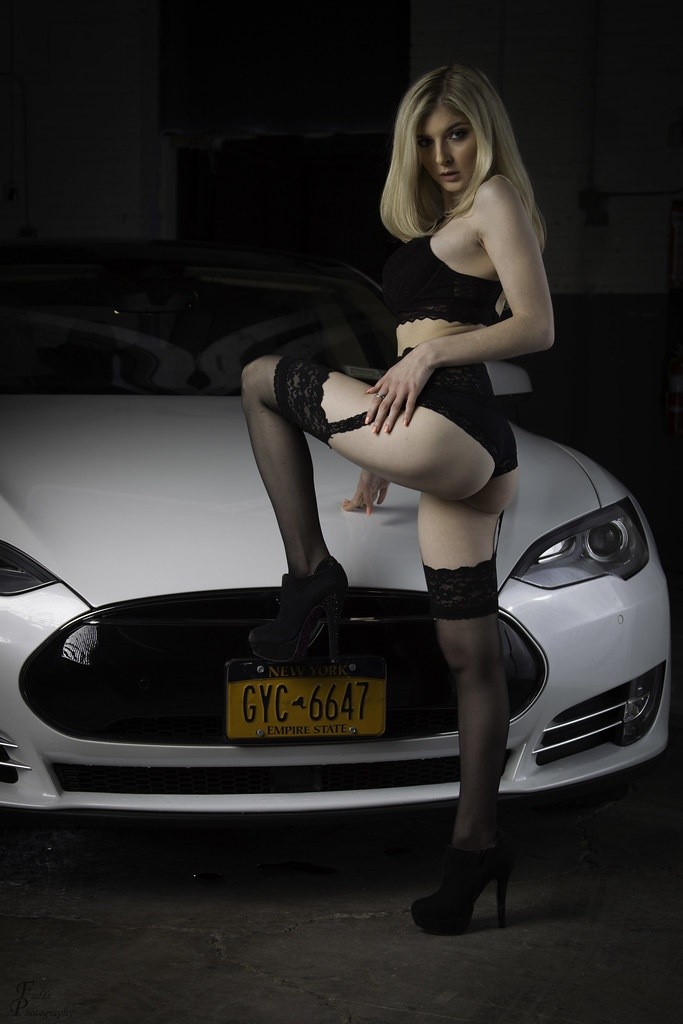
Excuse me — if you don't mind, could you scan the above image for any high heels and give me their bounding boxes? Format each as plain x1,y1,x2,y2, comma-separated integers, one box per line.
248,556,349,666
410,839,514,934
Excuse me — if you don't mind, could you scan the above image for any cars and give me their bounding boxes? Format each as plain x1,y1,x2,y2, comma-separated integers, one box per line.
0,249,675,820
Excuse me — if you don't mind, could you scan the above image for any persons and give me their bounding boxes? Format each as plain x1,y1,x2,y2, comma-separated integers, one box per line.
240,62,556,934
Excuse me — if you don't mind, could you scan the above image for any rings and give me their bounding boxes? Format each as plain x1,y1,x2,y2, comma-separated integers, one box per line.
375,394,385,400
